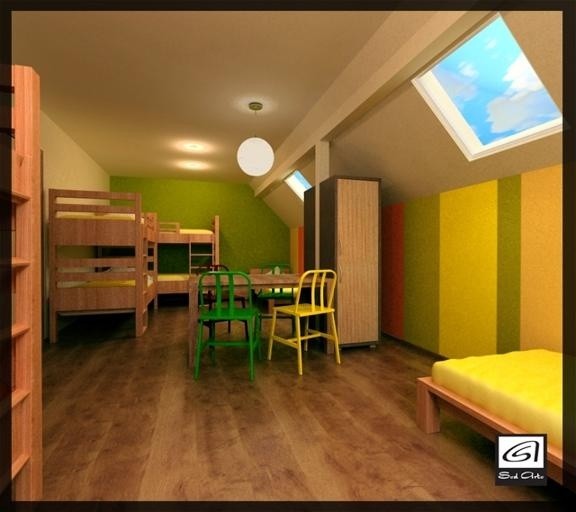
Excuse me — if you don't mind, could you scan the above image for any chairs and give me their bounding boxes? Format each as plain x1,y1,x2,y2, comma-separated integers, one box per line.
188,263,343,383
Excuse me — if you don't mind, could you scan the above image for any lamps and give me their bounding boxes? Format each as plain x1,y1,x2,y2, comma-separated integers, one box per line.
236,100,275,178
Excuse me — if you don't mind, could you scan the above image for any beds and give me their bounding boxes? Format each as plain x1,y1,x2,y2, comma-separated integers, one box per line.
46,187,222,345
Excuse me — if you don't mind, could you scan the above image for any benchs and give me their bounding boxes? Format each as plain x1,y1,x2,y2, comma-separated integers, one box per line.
415,347,565,485
